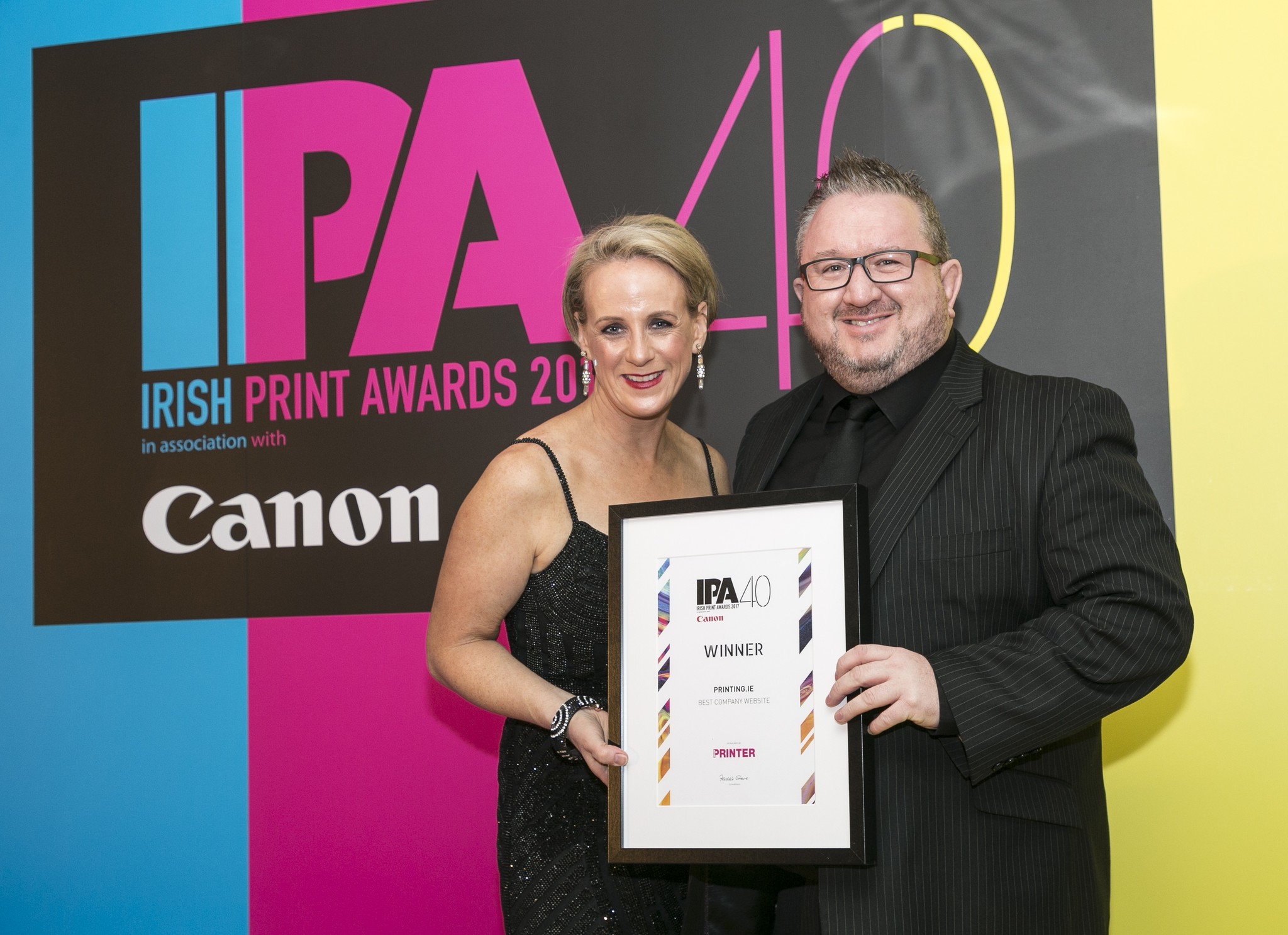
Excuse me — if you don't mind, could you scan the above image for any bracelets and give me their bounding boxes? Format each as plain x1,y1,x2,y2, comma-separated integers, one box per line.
549,689,601,766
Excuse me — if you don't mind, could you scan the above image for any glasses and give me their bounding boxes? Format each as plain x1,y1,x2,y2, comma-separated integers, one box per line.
800,250,941,291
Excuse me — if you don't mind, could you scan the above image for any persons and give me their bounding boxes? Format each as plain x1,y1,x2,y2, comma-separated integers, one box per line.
736,143,1196,935
425,211,760,934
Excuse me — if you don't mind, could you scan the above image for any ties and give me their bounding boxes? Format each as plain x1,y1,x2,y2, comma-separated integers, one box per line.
814,398,877,488
